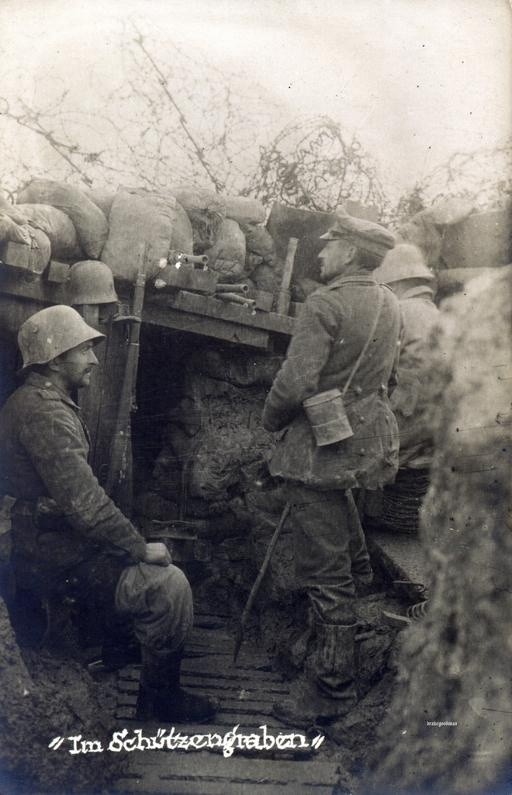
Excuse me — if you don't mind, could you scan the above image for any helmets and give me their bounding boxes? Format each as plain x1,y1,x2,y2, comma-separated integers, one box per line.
373,242,437,286
15,303,108,378
66,259,120,308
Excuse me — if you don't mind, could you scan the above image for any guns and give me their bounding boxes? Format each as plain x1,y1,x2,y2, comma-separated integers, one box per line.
95,242,152,522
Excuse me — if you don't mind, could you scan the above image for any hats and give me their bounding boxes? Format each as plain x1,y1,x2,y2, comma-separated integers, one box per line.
316,214,399,260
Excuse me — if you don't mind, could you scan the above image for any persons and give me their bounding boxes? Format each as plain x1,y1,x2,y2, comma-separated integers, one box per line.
377,240,454,460
259,214,405,729
2,303,223,723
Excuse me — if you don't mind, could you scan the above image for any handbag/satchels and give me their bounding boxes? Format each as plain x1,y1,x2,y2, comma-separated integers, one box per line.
300,389,355,450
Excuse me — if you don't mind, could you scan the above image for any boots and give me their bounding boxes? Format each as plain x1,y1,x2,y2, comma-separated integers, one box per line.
270,616,383,726
133,643,218,727
